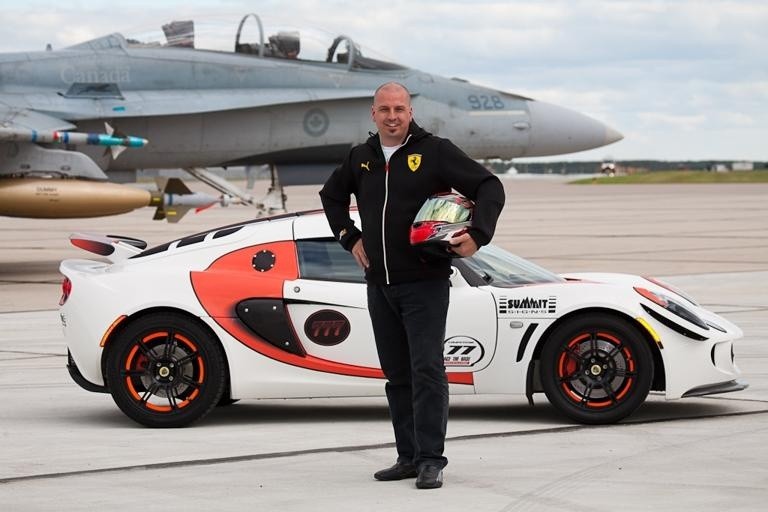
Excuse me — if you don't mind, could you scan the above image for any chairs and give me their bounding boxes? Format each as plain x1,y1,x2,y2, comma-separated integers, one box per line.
308,242,333,278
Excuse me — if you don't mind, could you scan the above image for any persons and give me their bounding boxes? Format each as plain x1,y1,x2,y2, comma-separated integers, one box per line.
317,80,507,490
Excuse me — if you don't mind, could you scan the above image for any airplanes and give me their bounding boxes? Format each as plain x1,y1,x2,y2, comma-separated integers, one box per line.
0,13,625,218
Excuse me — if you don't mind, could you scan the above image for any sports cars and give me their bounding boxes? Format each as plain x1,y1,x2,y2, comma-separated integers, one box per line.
59,206,749,428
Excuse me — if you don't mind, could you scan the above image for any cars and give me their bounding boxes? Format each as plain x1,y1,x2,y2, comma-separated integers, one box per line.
601,160,615,173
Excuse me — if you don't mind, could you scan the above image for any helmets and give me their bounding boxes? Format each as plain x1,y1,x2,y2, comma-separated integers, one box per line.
408,192,476,247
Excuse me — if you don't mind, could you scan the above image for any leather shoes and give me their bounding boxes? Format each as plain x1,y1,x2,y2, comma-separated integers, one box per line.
416,464,443,489
374,460,420,480
